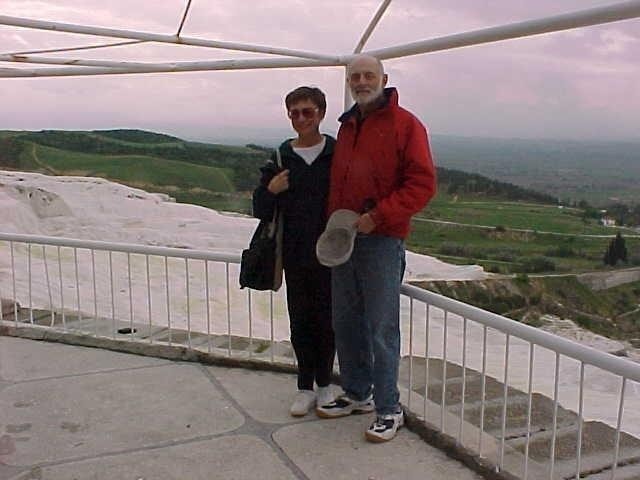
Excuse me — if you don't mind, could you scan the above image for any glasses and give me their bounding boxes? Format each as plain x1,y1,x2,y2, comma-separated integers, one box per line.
287,107,320,118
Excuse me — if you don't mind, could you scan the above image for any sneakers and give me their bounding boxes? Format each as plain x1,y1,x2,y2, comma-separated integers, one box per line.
289,390,374,417
365,410,404,442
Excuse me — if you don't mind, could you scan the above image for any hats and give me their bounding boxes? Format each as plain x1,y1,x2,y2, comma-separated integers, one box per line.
315,208,360,268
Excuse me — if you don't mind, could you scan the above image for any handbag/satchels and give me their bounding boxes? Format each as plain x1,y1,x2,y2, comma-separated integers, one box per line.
239,200,283,291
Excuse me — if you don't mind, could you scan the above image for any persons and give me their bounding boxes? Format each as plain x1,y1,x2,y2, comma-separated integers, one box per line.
253,87,337,416
315,55,436,442
